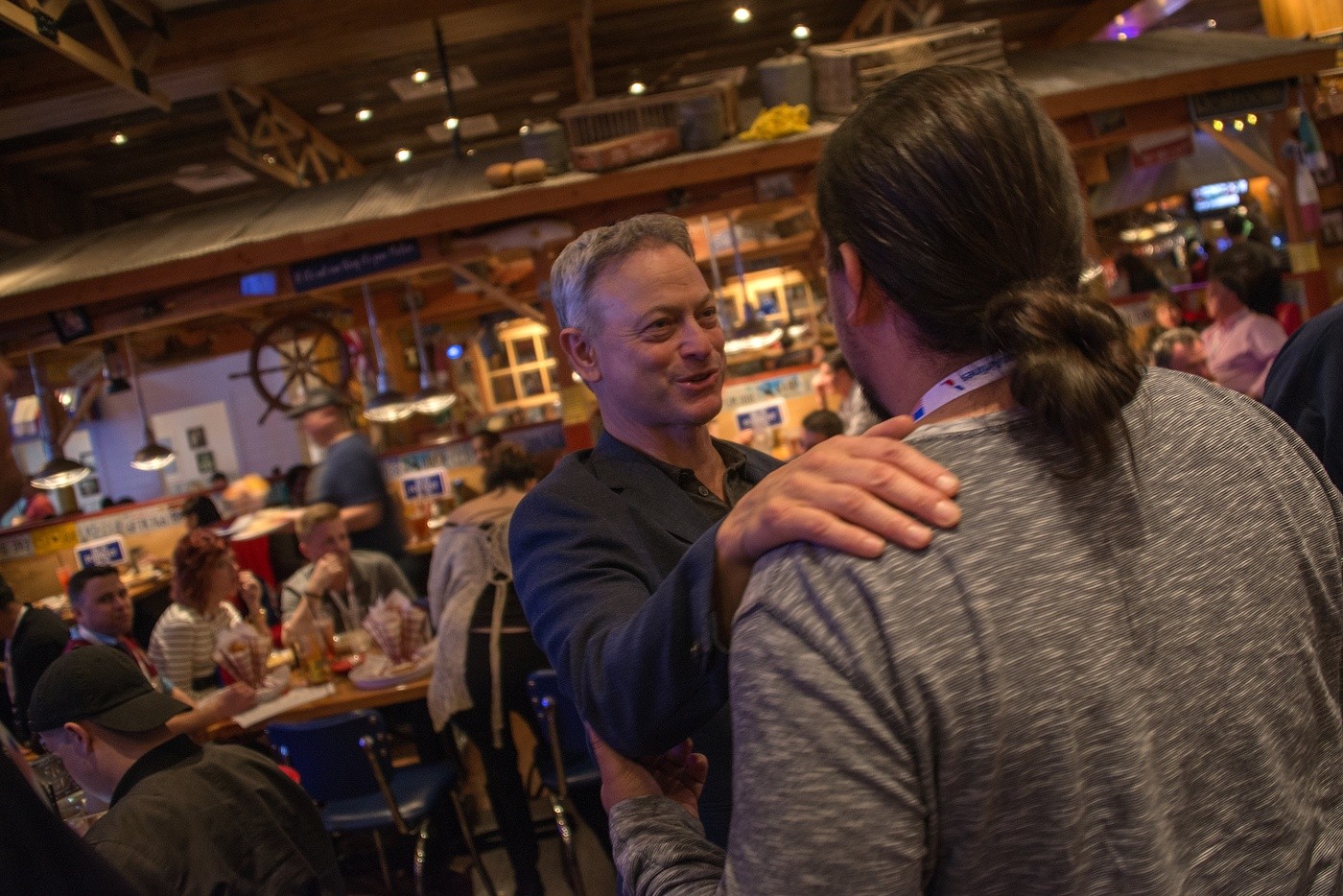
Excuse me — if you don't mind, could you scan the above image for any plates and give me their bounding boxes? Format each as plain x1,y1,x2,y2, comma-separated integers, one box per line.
334,653,365,671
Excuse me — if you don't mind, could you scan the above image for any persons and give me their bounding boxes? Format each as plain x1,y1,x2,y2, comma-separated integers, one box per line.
4,359,423,604
422,447,629,895
0,575,72,736
811,352,883,437
785,409,846,457
450,426,505,500
40,645,383,896
1,726,147,896
142,529,277,703
281,500,483,856
1096,198,1343,493
508,209,963,858
56,565,265,746
578,60,1340,896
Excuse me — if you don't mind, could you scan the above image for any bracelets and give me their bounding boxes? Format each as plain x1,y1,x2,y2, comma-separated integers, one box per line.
303,590,324,601
247,606,268,622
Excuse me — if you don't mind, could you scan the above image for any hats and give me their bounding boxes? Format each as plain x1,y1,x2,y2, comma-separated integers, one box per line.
29,645,194,732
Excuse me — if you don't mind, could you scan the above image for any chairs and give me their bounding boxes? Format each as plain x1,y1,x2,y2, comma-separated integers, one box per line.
1,498,533,896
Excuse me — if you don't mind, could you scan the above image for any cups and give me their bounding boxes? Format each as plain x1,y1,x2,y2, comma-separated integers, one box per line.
284,607,338,690
